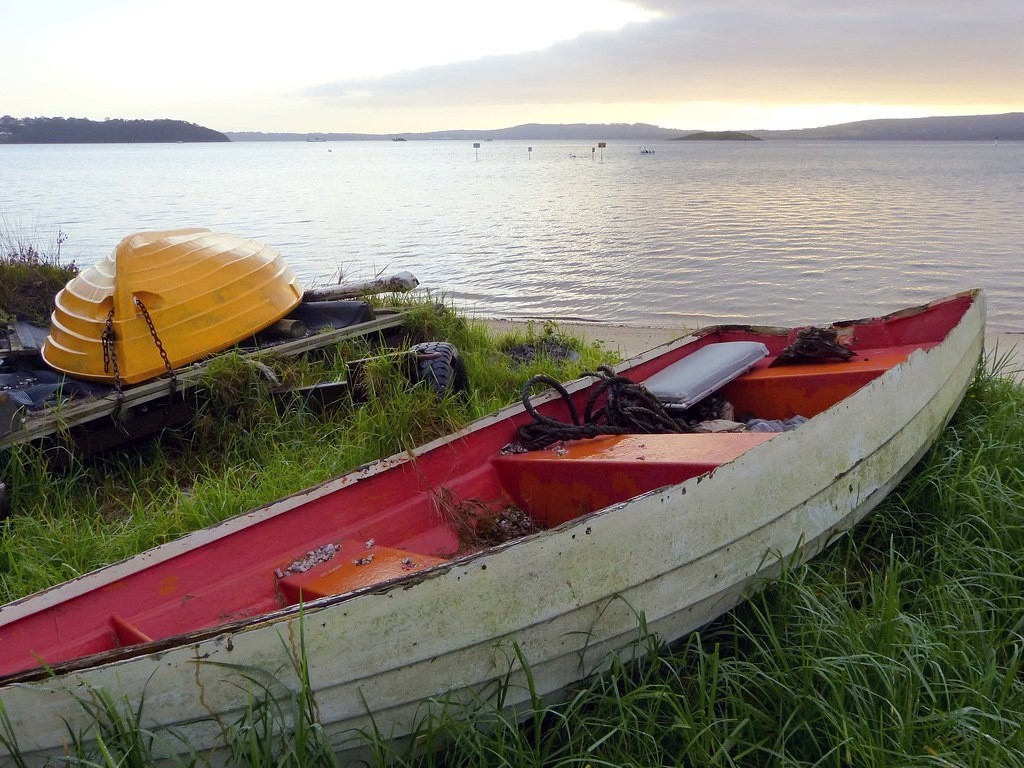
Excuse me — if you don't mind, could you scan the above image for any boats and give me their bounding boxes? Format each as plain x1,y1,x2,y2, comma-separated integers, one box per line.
1,284,991,768
40,227,305,389
